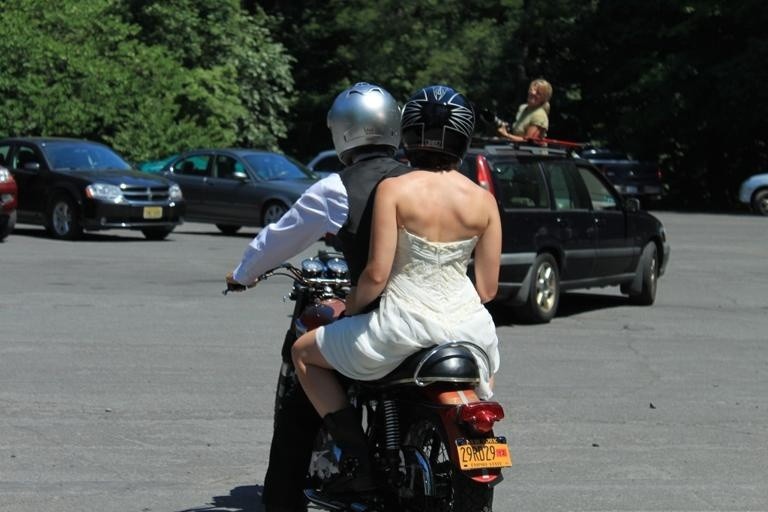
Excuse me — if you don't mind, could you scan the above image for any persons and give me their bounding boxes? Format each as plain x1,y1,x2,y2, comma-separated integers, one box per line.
226,82,412,512
289,85,505,499
495,77,553,148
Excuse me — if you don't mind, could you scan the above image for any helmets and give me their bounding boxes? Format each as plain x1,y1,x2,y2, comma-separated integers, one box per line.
401,85,477,166
327,82,402,168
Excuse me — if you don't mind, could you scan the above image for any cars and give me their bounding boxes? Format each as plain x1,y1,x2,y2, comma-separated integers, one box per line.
0,162,18,241
738,172,768,216
153,148,323,235
577,146,647,211
303,150,349,178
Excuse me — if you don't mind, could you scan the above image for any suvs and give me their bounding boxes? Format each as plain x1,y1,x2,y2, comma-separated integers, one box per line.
0,137,185,241
456,142,671,324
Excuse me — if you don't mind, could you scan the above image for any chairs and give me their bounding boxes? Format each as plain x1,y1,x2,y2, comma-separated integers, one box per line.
181,161,194,174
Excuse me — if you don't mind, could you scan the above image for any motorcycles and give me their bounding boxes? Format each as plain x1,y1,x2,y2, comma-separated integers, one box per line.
221,250,513,512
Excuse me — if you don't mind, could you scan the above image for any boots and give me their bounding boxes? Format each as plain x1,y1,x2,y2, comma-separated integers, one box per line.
319,402,384,495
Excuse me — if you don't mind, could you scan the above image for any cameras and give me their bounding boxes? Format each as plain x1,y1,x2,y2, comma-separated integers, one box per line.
493,116,512,132
